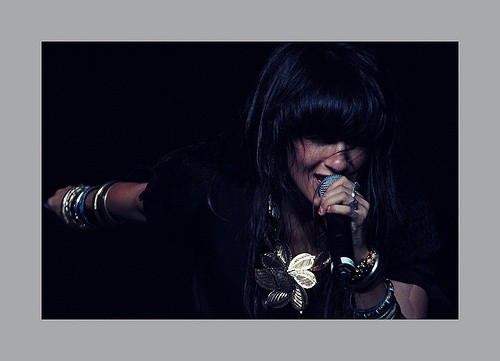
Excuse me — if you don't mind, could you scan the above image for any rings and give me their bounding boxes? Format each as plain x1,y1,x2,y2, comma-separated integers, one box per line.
342,196,359,210
351,189,357,198
354,181,360,191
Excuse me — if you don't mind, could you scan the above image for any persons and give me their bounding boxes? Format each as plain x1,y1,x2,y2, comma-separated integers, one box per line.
42,42,442,319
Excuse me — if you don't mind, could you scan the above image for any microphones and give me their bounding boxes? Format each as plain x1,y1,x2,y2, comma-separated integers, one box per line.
318,175,355,276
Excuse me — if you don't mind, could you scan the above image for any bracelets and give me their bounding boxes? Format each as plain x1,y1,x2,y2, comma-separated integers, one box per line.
60,179,126,235
333,242,407,320
